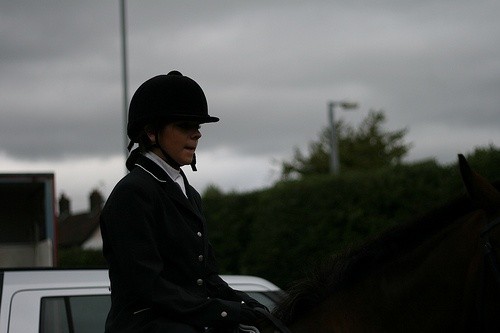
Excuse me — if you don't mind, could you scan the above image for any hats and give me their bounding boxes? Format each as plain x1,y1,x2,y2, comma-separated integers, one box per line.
127,71,219,139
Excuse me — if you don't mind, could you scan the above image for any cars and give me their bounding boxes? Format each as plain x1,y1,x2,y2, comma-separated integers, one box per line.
0,266,293,333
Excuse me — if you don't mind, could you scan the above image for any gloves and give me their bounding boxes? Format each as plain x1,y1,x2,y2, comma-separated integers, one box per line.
222,302,270,325
239,292,271,313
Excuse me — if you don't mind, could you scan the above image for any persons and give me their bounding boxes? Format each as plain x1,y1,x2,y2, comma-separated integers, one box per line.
99,71,270,333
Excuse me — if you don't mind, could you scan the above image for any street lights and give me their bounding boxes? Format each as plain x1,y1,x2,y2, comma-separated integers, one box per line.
327,99,358,175
